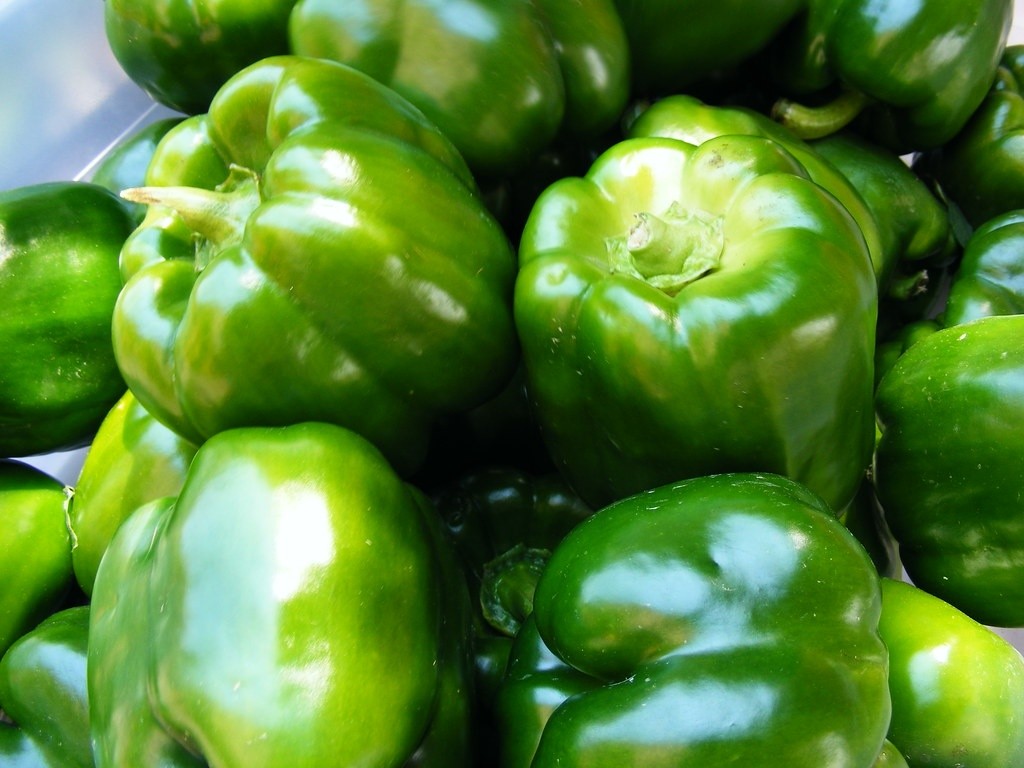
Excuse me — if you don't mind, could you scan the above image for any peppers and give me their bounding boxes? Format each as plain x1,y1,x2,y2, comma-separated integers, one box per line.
0,0,1024,767
